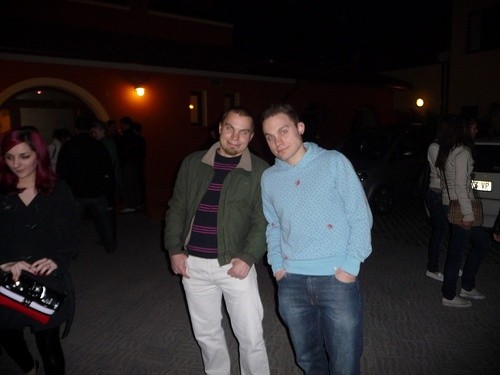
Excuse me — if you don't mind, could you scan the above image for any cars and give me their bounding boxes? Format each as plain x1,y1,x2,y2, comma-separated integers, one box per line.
471,142,500,244
339,123,430,215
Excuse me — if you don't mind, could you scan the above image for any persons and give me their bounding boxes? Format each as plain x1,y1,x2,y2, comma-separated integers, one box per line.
423,106,500,308
0,116,145,375
165,105,272,375
260,104,373,375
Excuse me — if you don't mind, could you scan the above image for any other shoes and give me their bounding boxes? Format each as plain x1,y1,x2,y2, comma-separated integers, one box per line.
459,287,485,298
443,296,472,308
426,271,444,281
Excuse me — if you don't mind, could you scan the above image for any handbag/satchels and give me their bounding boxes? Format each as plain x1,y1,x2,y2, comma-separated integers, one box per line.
0,271,63,325
448,199,483,226
